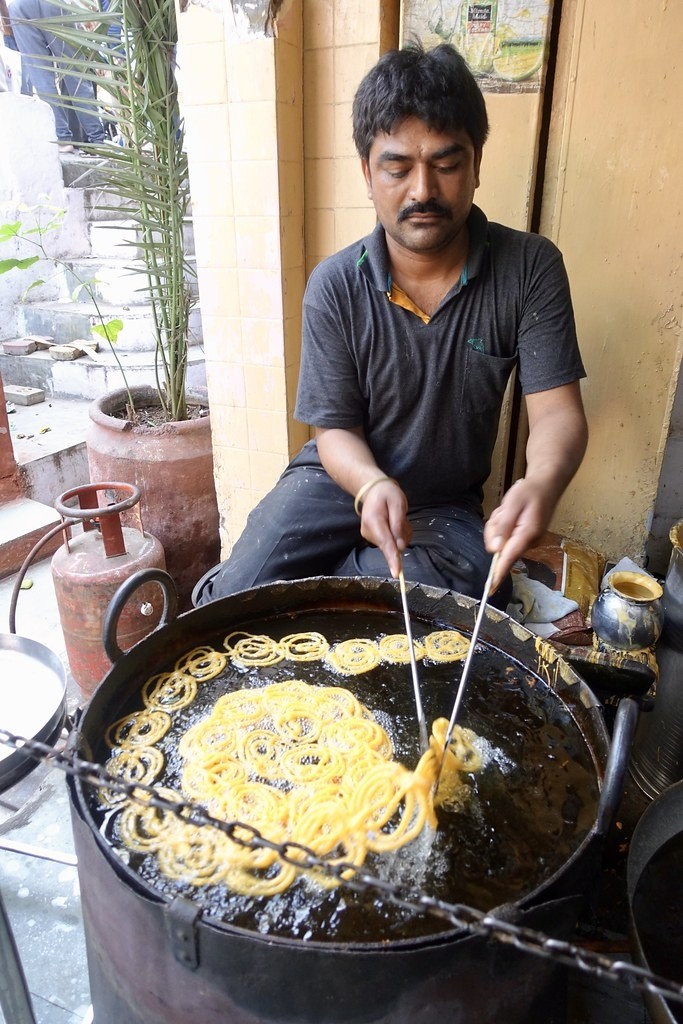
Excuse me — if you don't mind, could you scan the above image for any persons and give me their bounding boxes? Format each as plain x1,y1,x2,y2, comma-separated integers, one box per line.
0,0,126,158
191,42,588,610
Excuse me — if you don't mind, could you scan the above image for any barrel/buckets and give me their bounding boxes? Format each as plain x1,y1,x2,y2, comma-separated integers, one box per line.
64,775,621,1024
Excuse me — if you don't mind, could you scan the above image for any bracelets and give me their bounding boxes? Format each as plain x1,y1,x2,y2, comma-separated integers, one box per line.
355,476,401,516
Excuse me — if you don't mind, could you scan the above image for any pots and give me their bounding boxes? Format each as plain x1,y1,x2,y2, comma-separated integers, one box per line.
628,780,683,1024
71,567,634,949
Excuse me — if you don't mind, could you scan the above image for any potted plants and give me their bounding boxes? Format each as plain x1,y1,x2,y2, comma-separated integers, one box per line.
0,0,221,618
